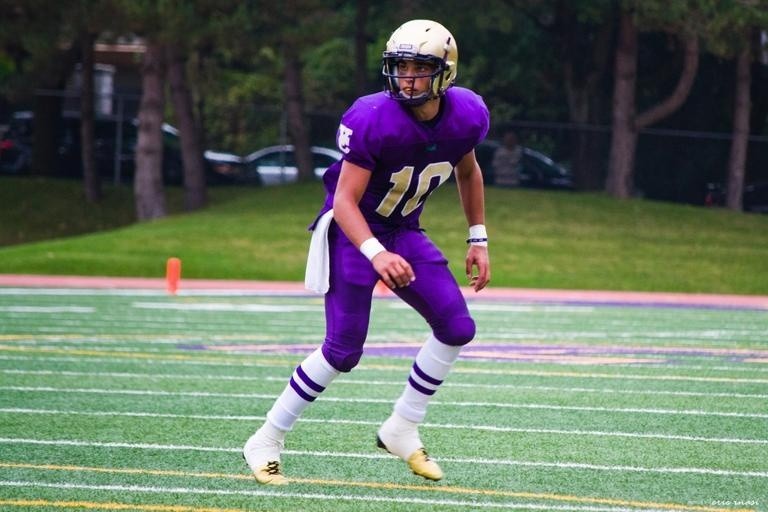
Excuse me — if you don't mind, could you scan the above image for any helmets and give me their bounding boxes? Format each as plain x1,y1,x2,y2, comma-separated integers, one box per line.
379,16,462,109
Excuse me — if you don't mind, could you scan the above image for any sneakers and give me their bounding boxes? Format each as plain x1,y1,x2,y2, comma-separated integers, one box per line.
240,437,288,487
376,428,444,483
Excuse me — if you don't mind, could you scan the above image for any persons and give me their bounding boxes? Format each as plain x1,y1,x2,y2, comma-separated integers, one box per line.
240,18,493,489
490,123,528,190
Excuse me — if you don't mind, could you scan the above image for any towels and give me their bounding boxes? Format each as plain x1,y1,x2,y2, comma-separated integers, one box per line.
304,209,334,294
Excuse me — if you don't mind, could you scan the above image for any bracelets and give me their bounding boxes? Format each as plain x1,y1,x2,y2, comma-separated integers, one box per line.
359,237,385,260
465,237,489,243
468,224,488,248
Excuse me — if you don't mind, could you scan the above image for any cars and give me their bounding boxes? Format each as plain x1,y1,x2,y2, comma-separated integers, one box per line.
474,139,577,191
245,144,344,185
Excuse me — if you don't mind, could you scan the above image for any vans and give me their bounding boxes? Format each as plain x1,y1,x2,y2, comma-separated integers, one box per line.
0,108,262,186
